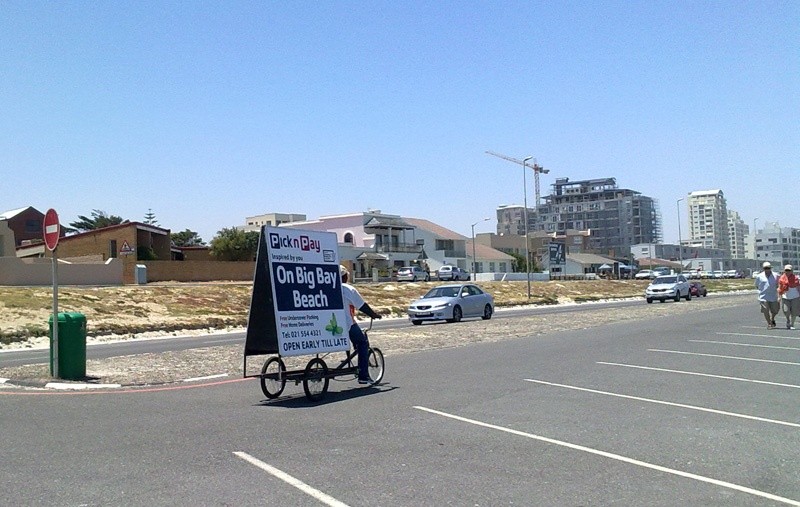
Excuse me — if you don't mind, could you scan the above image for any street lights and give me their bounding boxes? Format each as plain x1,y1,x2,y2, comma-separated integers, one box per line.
523,156,533,299
471,218,492,281
677,198,686,274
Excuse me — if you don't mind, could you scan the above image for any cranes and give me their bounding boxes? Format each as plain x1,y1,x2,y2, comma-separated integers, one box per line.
484,150,550,229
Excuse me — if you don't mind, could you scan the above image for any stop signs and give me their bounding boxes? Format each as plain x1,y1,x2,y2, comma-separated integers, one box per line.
42,208,60,251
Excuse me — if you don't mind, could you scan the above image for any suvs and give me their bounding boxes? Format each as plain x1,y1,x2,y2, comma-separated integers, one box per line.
645,273,692,304
396,266,429,282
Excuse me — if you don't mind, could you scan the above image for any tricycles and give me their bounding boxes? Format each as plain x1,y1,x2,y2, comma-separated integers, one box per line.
261,315,385,405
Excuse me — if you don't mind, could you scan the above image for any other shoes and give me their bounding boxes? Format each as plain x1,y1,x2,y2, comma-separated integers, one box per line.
786,320,791,329
790,324,795,330
358,377,375,383
767,322,773,329
772,320,776,327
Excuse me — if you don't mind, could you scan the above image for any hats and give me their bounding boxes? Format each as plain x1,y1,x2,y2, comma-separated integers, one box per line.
339,264,350,276
763,262,772,268
784,264,793,270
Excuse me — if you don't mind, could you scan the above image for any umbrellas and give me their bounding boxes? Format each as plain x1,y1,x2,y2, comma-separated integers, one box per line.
599,264,612,274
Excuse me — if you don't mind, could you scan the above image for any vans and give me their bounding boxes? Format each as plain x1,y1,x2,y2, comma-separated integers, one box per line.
653,267,670,276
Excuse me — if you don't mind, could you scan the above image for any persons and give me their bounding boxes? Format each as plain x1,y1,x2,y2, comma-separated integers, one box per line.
339,265,382,384
423,263,431,282
755,262,781,329
777,264,800,329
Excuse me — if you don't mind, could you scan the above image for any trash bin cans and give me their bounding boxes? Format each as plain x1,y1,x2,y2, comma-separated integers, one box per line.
49,313,87,381
135,264,147,284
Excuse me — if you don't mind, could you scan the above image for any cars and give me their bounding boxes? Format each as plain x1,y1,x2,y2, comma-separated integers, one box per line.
680,268,738,280
407,283,495,325
438,265,470,281
752,271,761,279
635,269,655,280
689,282,708,298
